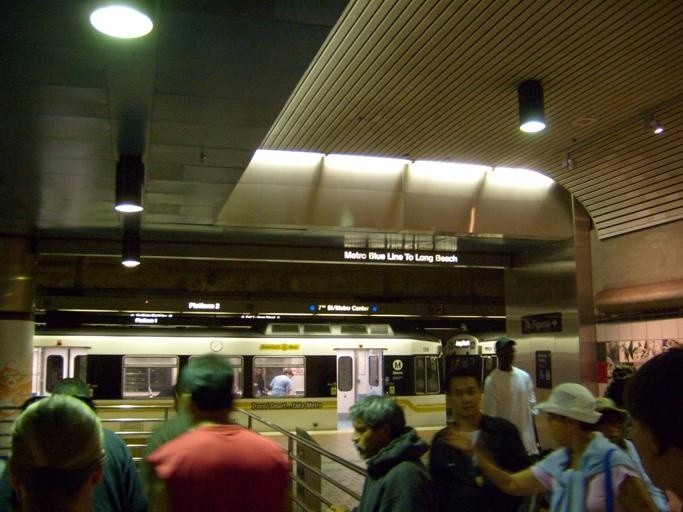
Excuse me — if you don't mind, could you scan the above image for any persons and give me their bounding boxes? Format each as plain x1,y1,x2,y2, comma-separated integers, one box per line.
0,376,150,512
330,336,682,512
269,368,294,395
254,368,268,396
137,351,295,512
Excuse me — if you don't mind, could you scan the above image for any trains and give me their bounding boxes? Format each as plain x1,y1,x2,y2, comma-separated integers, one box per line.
31,322,503,434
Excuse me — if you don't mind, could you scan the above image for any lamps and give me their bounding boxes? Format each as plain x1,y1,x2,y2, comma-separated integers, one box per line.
518,80,546,133
113,160,145,268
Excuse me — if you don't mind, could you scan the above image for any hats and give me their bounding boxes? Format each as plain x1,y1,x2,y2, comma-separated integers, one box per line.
595,397,628,422
533,382,603,424
495,336,516,350
